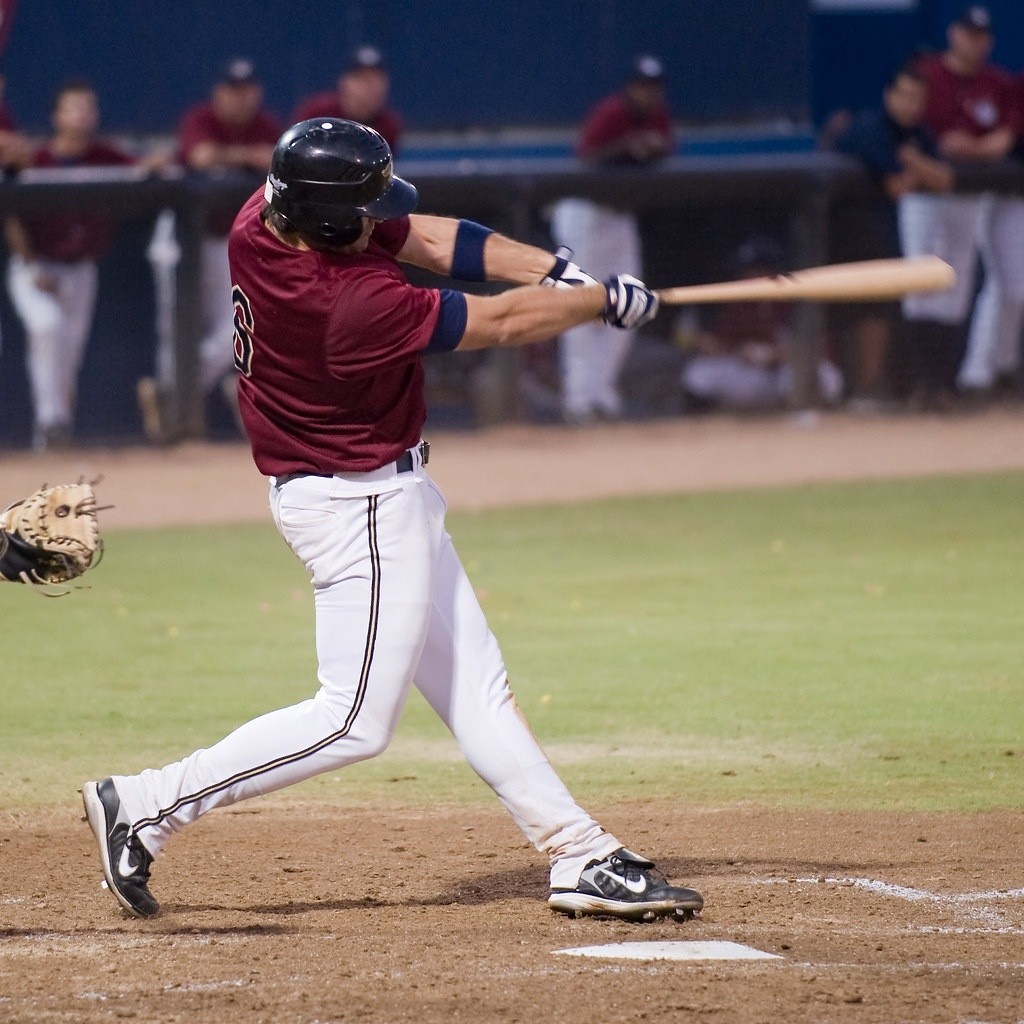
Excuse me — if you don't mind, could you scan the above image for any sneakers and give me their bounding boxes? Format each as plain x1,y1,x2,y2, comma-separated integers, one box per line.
548,848,705,920
78,777,160,918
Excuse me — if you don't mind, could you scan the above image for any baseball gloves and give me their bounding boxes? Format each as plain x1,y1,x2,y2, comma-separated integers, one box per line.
0,481,106,589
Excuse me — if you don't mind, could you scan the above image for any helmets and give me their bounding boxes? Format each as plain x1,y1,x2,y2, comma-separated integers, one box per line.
264,117,419,247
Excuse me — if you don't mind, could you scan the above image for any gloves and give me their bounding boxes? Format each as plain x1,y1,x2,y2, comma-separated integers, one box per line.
602,273,659,332
542,246,602,289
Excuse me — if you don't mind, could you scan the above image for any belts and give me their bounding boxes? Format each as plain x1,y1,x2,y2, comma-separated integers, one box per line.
276,442,430,487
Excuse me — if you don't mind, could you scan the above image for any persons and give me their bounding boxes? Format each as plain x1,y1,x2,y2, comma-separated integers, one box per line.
0,3,1024,456
0,473,115,597
77,116,706,922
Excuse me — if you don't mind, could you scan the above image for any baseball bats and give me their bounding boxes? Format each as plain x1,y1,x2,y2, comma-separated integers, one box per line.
653,254,963,308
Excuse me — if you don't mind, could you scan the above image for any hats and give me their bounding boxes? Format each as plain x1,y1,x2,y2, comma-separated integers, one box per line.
956,7,995,32
631,58,665,86
221,58,258,85
348,47,386,74
